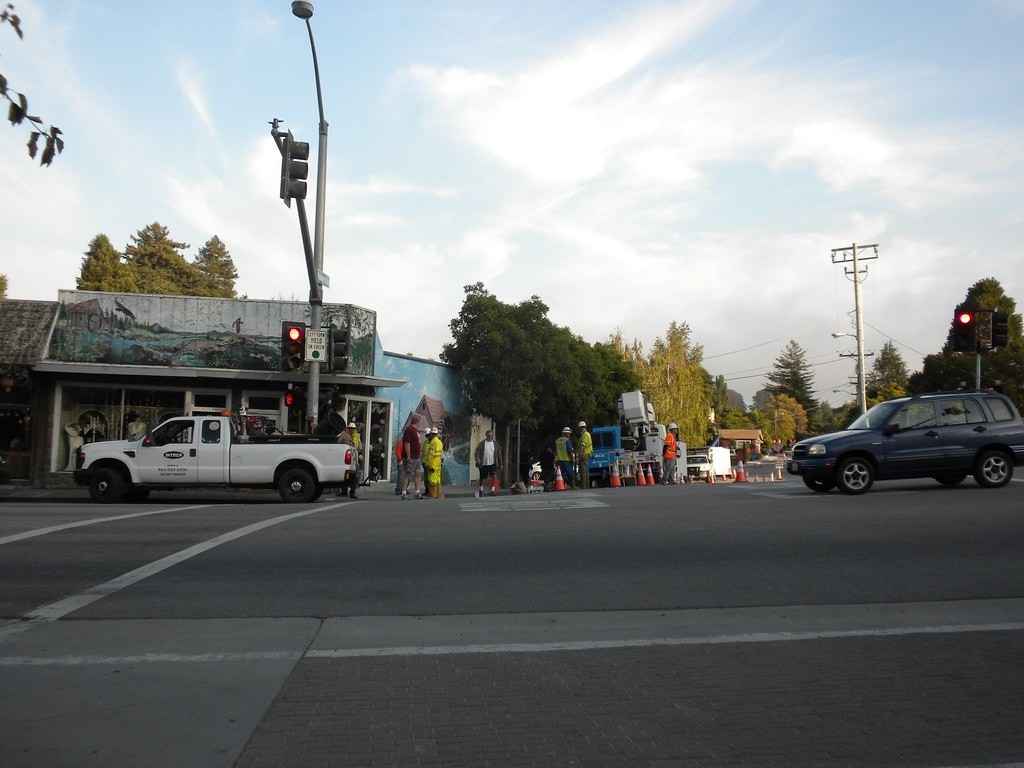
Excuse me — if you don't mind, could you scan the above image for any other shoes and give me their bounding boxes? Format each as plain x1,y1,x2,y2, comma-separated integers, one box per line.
661,479,666,485
672,482,679,485
479,490,483,497
401,494,408,500
414,492,423,499
335,493,348,497
490,491,496,496
350,494,358,498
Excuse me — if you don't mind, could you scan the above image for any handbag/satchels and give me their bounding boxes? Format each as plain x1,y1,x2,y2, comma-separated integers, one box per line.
396,439,403,462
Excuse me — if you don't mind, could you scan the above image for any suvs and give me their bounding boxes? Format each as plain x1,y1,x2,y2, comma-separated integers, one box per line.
787,389,1024,494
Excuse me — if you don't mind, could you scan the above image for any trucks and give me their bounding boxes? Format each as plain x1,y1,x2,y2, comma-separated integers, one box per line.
686,446,732,481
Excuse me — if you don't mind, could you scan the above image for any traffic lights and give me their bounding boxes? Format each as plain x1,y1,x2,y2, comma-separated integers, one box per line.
286,389,298,407
329,324,350,372
281,320,306,372
954,308,977,352
991,310,1009,349
278,129,309,208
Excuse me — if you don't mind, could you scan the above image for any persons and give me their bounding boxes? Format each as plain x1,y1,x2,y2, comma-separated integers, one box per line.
573,421,593,490
661,423,680,485
421,427,443,499
401,415,423,500
358,422,381,476
553,427,581,490
128,418,147,435
395,439,410,495
336,422,362,499
10,434,24,448
474,430,503,497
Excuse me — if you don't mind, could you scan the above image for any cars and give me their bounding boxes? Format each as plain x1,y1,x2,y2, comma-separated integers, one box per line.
528,461,543,480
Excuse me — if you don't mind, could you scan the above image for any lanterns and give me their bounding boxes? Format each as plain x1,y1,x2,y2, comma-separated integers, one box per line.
0,374,16,393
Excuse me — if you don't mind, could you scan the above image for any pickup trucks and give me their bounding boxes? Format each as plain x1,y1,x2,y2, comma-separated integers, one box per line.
72,416,360,503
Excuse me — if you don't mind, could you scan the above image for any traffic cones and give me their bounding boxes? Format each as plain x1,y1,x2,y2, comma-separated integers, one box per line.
711,470,716,483
638,463,646,485
658,466,665,484
732,458,750,483
611,461,621,487
768,473,774,482
721,472,727,481
646,464,656,486
776,468,782,480
783,453,786,459
554,466,567,491
676,473,693,485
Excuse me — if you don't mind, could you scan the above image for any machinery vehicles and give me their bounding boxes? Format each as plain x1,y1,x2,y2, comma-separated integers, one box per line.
588,390,687,484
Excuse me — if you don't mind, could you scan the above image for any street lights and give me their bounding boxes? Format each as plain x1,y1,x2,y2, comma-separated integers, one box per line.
292,0,328,433
832,332,869,428
833,390,858,406
774,412,785,437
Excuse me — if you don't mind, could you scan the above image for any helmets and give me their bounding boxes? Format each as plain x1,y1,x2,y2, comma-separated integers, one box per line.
578,421,587,427
562,427,572,433
431,427,438,434
669,423,678,429
346,422,356,428
424,428,431,436
